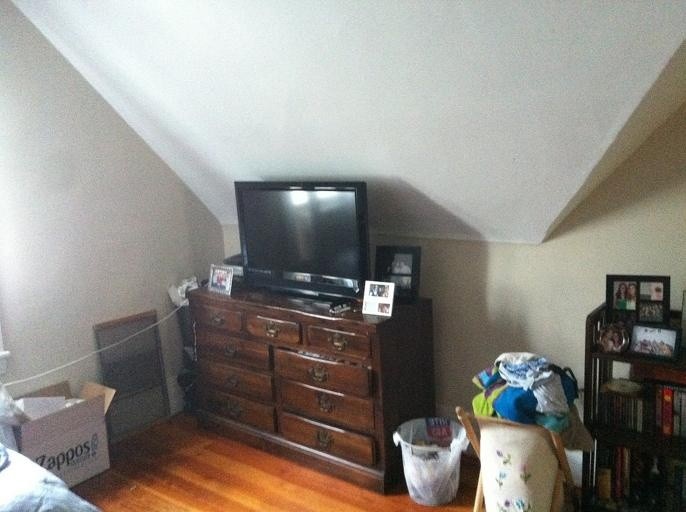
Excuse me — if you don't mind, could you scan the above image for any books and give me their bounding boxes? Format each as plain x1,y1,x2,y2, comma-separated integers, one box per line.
596,361,686,512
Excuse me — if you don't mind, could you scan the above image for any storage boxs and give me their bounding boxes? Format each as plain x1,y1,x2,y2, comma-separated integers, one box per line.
11,380,117,489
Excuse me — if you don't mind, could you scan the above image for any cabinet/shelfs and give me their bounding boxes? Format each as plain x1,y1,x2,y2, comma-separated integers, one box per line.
581,300,686,511
186,285,436,494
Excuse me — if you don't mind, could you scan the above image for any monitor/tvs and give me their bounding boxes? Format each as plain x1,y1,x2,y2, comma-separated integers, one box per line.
233,179,370,304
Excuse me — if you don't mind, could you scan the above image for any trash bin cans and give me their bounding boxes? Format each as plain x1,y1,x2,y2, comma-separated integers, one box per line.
396,416,468,507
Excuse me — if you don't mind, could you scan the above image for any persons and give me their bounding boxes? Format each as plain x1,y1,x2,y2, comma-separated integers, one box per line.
633,329,672,359
627,283,637,311
651,286,664,302
602,329,614,353
213,270,229,291
612,331,624,353
368,259,412,314
615,283,629,309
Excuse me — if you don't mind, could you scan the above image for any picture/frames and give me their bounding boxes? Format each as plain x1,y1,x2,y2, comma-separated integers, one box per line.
208,264,234,297
361,245,422,317
606,274,671,329
